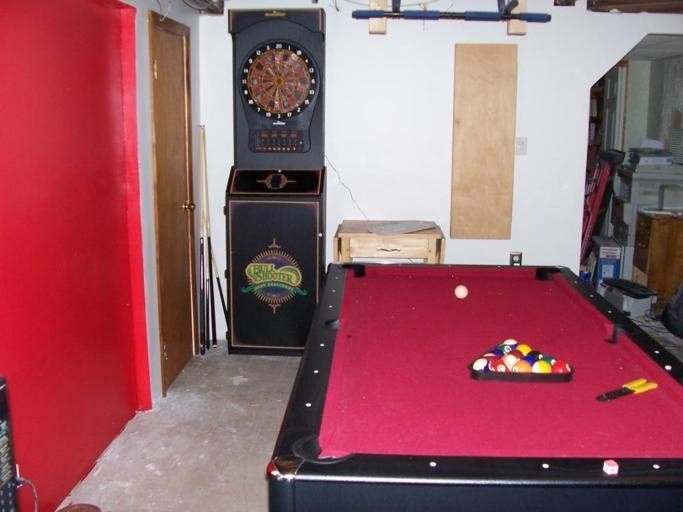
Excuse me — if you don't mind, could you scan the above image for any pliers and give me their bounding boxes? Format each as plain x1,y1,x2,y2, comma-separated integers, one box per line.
595,377,658,402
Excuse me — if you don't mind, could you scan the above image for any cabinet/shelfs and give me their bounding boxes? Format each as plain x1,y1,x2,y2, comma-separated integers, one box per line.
612,168,683,279
633,209,683,314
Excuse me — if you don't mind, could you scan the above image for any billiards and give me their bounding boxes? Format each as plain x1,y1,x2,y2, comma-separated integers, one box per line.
472,339,572,375
454,286,468,297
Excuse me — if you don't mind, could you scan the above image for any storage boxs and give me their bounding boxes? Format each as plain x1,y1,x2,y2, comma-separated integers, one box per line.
592,236,621,259
593,259,620,288
601,283,651,317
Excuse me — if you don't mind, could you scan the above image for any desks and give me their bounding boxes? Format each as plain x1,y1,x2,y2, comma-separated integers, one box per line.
333,221,446,265
265,264,683,512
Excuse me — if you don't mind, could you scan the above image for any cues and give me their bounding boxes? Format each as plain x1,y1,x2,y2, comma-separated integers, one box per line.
199,126,228,354
